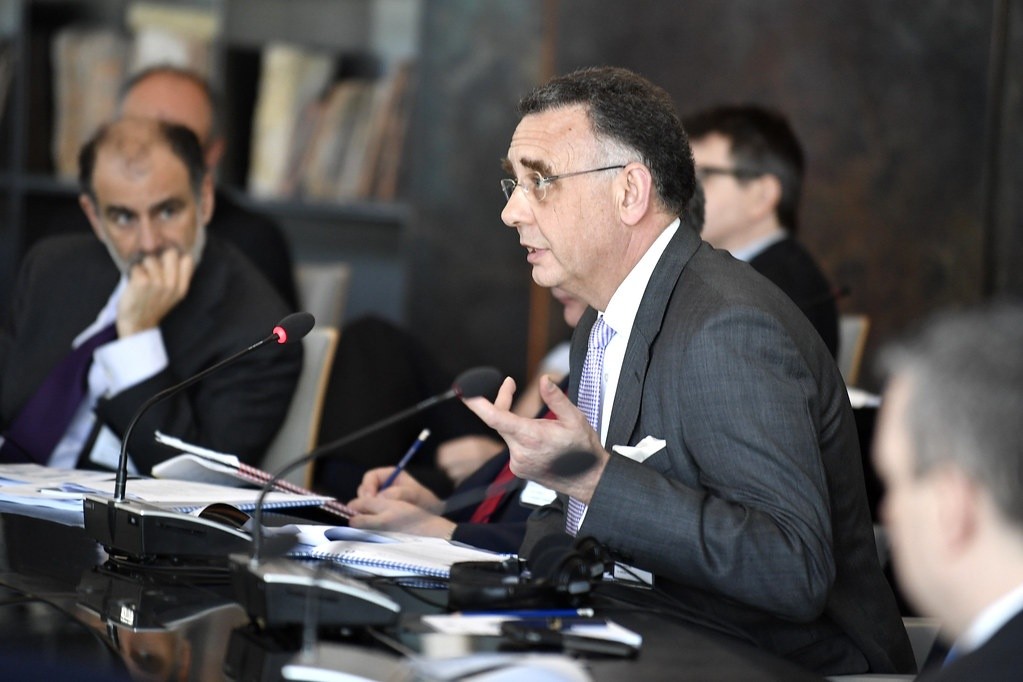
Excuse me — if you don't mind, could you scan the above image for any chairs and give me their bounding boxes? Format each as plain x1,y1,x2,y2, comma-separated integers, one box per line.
252,325,340,487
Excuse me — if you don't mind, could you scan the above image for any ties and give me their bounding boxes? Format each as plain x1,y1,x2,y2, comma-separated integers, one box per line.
5,324,119,464
565,316,618,539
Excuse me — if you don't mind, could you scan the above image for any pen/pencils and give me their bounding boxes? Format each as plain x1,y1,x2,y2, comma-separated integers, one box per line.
380,428,431,492
451,606,594,618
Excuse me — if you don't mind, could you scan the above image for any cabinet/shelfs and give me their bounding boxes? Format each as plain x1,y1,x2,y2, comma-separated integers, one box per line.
0,0,419,318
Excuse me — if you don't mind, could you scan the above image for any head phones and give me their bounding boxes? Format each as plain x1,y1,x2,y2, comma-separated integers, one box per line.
448,532,613,612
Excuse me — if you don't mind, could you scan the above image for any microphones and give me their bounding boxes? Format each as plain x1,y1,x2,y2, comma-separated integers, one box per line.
81,312,601,682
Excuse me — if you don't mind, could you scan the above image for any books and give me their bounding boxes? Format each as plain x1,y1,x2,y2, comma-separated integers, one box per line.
0,463,335,557
315,538,518,590
148,429,356,520
52,4,423,202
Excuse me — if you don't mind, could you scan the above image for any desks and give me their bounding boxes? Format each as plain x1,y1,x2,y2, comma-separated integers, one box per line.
0,512,823,682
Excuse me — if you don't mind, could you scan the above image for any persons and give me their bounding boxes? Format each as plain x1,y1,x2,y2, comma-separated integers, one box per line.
431,343,571,503
347,376,570,555
1,116,305,476
111,63,306,310
872,304,1023,681
460,67,916,677
438,104,840,489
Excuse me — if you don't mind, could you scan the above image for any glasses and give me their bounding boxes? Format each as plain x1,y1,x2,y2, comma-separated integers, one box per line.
500,165,626,203
695,163,751,183
